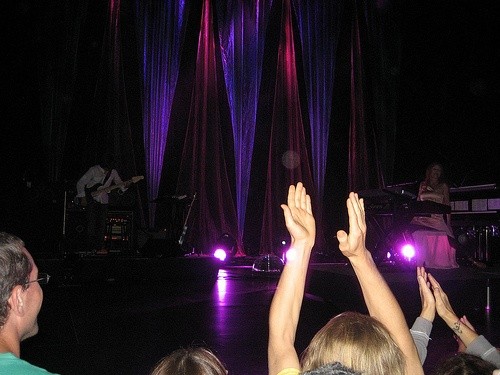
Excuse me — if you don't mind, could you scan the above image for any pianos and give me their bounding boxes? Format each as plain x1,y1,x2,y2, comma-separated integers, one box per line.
354,200,451,273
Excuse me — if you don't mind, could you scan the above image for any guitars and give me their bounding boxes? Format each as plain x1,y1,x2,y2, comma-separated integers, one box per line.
77,176,145,210
177,191,198,246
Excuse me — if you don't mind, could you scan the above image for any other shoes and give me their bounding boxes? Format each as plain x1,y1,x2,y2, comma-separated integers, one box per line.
95,250,108,255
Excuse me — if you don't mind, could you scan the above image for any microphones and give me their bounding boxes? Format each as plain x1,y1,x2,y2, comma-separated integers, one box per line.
416,176,423,183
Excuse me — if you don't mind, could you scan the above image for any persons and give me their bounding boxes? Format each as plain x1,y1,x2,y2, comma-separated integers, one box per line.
410,266,500,375
149,346,228,375
411,159,454,238
75,152,128,254
267,182,425,375
0,232,59,375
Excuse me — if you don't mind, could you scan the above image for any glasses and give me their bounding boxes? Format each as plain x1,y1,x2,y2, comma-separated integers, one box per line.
20,274,51,285
